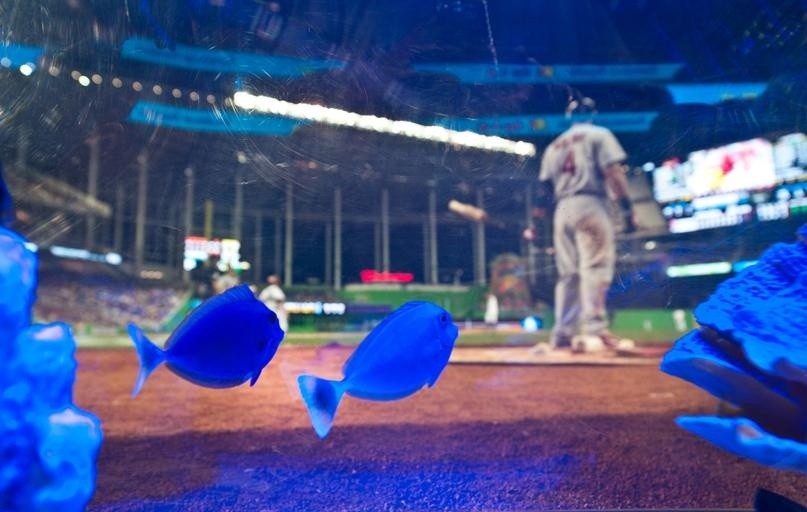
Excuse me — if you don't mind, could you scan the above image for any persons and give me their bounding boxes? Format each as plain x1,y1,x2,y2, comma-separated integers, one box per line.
536,97,640,349
189,253,289,334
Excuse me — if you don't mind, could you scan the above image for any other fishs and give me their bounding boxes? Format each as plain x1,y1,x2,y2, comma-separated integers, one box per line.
296,299,459,441
127,285,285,399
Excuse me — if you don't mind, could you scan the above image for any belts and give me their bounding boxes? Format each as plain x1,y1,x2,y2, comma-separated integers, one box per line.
574,190,604,198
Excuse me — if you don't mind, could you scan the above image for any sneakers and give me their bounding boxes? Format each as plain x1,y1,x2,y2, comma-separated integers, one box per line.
598,331,635,352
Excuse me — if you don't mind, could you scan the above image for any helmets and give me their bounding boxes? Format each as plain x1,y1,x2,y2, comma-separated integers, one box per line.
565,97,595,122
